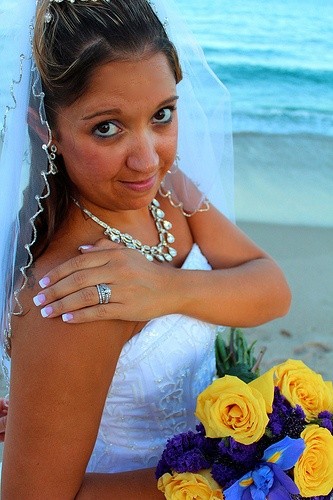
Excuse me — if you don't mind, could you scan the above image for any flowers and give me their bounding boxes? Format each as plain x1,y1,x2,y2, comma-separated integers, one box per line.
152,358,333,500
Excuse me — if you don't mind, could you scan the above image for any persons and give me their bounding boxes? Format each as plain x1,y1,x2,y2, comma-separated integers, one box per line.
1,0,292,500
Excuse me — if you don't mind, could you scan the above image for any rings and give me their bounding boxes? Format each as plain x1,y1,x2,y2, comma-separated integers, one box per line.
97,283,112,304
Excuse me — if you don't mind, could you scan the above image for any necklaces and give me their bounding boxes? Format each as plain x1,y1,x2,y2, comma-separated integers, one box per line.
68,188,177,264
